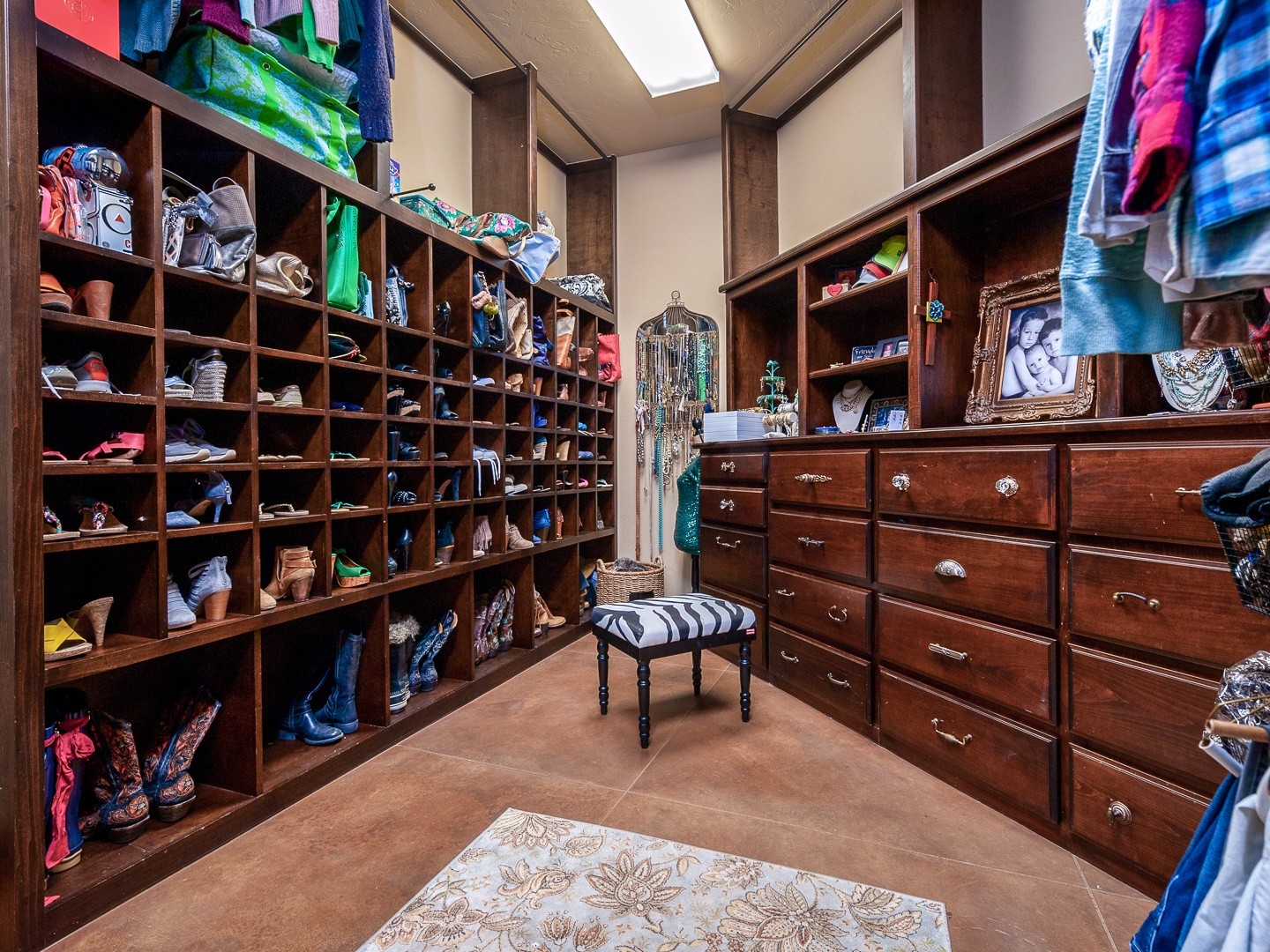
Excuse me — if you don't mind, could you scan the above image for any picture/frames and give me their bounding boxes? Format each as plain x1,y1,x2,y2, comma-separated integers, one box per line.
959,266,1097,425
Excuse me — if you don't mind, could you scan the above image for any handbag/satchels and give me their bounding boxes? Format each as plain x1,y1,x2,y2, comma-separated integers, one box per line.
39,127,623,382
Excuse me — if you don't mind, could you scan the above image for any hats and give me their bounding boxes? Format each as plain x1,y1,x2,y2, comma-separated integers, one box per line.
850,235,906,288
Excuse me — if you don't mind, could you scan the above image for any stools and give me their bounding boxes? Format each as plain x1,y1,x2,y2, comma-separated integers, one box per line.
587,590,759,749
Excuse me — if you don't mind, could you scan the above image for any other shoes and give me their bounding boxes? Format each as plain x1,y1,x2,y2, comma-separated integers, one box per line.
29,268,613,873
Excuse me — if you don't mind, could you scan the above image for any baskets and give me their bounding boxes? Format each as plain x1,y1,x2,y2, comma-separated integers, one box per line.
594,555,667,606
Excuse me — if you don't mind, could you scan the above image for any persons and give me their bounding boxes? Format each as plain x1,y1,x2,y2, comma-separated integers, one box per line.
1002,307,1078,399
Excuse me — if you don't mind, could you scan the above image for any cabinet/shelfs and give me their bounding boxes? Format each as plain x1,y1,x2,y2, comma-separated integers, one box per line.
3,8,618,952
696,101,1268,899
